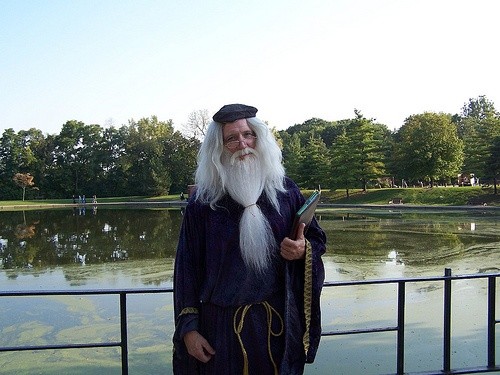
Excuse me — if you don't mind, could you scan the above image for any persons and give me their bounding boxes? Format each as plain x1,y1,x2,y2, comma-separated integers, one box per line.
72,195,97,203
181,208,185,215
180,192,184,201
172,104,326,375
72,206,97,216
384,172,480,188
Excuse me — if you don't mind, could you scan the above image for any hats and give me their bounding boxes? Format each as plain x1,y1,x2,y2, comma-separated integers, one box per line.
213,104,258,123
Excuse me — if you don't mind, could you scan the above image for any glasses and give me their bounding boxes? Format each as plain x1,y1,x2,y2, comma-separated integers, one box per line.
223,137,257,148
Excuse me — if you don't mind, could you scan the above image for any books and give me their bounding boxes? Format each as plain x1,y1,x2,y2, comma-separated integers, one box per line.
290,191,320,240
